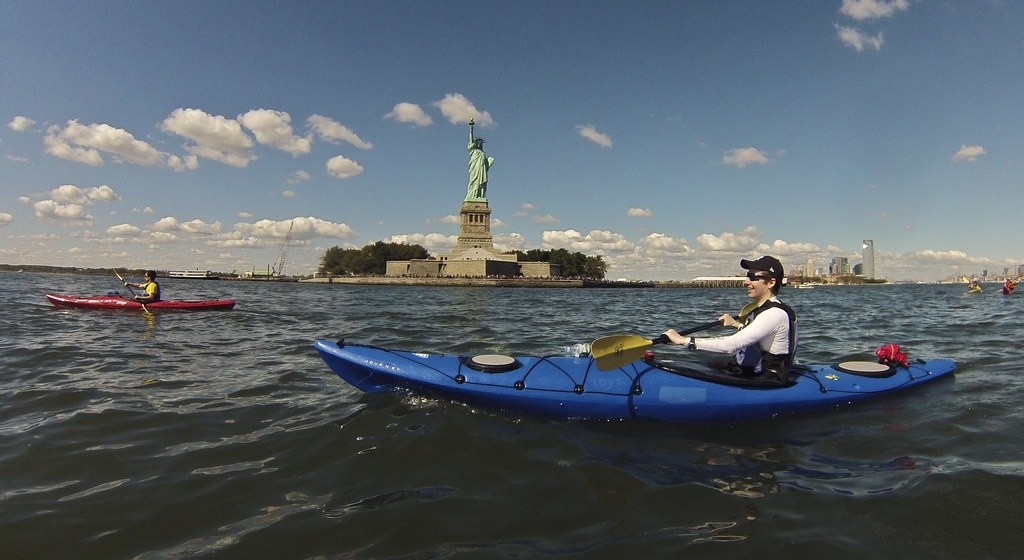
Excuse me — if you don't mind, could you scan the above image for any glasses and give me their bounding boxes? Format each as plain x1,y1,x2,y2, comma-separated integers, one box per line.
744,272,772,282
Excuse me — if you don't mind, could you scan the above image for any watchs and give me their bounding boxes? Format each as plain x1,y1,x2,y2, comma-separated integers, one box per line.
687,337,697,351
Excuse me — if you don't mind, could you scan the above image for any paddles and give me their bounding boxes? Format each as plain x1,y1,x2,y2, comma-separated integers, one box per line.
113,267,152,316
1012,273,1024,285
590,302,759,372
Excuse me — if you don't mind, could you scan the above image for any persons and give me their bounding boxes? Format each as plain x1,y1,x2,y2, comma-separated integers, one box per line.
463,124,495,203
661,256,798,379
124,270,160,303
968,281,982,293
1004,278,1013,289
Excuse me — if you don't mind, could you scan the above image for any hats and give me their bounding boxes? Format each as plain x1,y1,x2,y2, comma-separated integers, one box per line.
1006,278,1011,282
740,255,784,284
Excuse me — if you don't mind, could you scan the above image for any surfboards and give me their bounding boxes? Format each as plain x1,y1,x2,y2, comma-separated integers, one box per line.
969,290,978,293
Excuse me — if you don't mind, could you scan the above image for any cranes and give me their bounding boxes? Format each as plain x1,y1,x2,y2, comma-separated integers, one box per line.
275,220,295,276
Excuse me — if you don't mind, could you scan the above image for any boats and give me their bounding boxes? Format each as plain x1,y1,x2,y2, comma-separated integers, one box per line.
46,292,237,312
310,337,959,422
966,282,983,293
1002,275,1024,294
